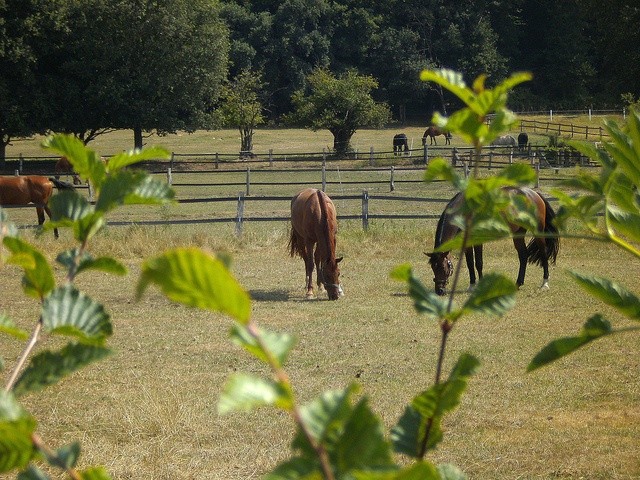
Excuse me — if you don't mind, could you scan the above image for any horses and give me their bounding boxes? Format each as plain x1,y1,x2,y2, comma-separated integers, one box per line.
0,176,62,240
518,132,528,153
285,188,344,300
490,135,518,151
423,181,559,296
421,126,452,146
392,134,408,156
55,154,108,184
